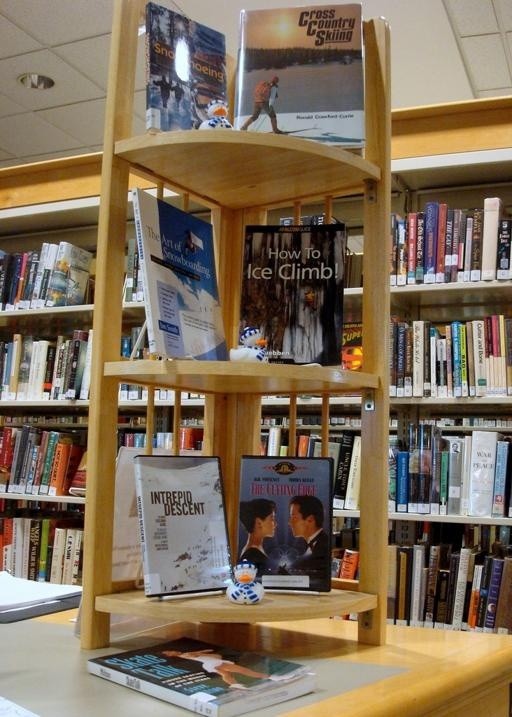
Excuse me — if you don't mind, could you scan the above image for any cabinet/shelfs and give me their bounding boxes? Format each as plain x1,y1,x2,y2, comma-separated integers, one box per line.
73,3,398,645
1,91,511,634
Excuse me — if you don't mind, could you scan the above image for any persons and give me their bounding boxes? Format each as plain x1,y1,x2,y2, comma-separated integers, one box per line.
241,74,284,135
170,81,187,104
159,648,297,690
151,75,172,108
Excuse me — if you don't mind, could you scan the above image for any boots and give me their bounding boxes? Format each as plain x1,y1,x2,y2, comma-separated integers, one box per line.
271,117,282,133
239,117,253,130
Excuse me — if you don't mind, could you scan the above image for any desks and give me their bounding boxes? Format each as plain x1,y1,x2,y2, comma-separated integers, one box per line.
0,608,512,717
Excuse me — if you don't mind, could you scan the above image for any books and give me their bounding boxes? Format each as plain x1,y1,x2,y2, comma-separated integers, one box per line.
234,2,367,152
87,634,317,715
143,0,231,136
1,191,512,636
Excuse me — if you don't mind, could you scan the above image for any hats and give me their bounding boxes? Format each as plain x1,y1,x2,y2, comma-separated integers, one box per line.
271,75,279,88
